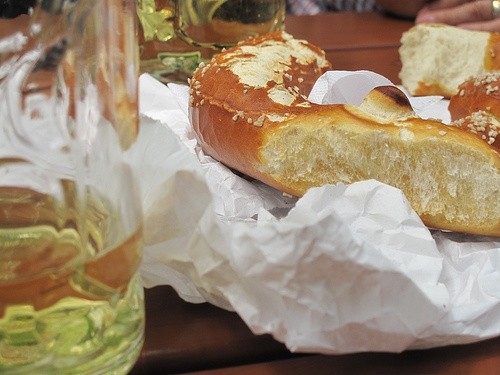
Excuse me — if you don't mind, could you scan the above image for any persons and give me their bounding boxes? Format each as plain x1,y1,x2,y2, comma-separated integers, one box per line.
373,0,500,34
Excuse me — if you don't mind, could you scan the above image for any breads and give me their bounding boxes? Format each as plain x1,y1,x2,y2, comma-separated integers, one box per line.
187,22,500,237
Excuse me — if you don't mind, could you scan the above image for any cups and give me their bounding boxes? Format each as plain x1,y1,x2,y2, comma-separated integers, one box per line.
172,0,286,50
0,0,146,375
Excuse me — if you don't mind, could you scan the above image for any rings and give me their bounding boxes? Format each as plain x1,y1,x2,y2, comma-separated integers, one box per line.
492,1,499,19
